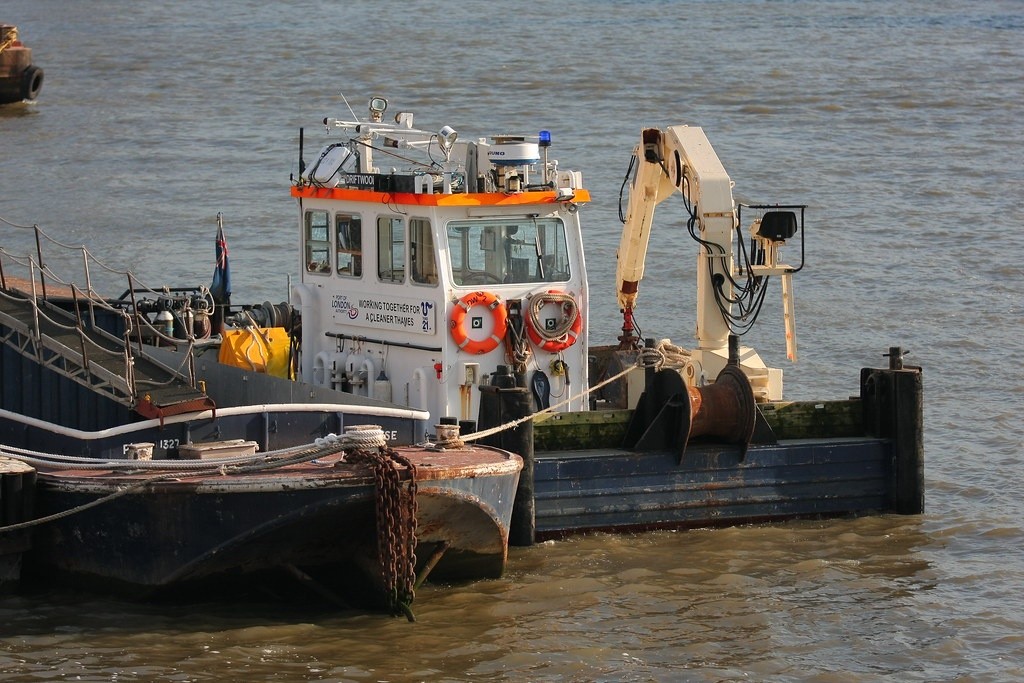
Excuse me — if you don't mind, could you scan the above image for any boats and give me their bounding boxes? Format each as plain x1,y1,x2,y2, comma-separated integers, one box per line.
0,25,42,107
0,213,525,621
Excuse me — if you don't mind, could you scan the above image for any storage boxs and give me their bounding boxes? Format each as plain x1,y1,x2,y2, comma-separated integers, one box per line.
302,145,356,188
177,441,258,460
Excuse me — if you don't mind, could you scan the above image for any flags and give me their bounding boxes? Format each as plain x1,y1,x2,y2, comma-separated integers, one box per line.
207,225,231,301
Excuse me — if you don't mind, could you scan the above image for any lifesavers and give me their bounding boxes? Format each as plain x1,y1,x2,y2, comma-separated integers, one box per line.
451,290,508,355
525,289,582,352
20,65,44,100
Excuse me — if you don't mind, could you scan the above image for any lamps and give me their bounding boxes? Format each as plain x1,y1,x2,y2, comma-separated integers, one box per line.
439,126,458,154
368,96,388,116
555,186,575,202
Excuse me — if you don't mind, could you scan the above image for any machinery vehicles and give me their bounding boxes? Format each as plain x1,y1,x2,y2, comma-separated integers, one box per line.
586,123,807,467
